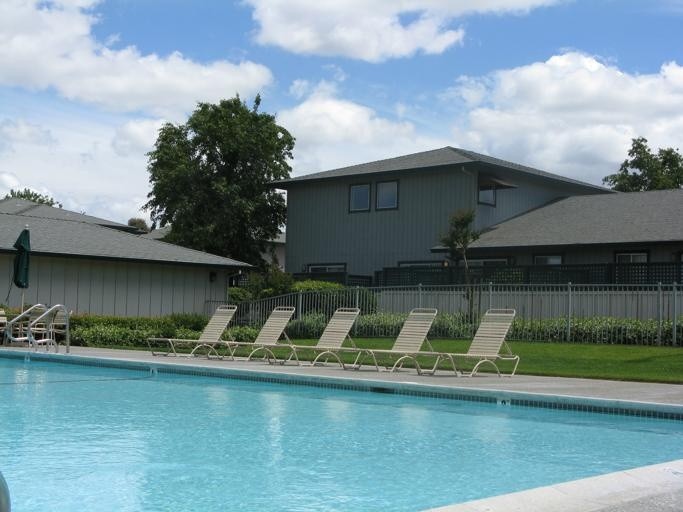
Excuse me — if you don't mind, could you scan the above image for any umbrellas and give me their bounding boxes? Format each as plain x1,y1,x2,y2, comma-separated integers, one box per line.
11,223,31,335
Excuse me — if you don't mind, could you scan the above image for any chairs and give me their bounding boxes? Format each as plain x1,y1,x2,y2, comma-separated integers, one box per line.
204,307,297,361
148,305,237,356
329,308,438,376
264,308,361,366
32,310,73,349
0,306,47,348
416,309,520,376
